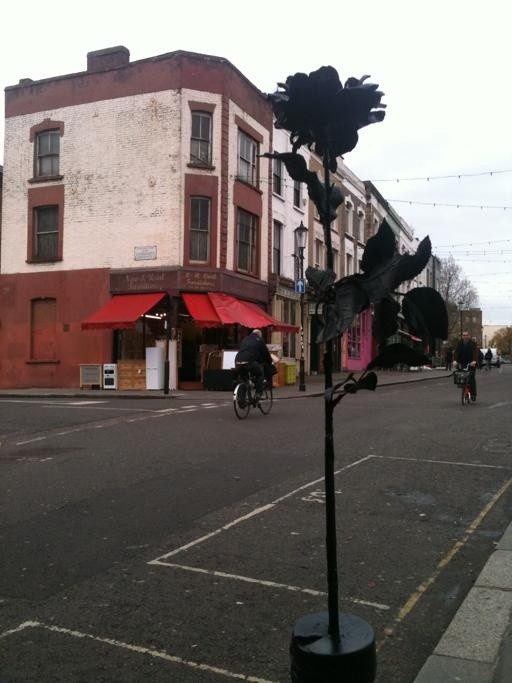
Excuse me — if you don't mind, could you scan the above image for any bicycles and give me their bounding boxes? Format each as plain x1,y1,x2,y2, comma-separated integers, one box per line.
453,362,472,407
233,375,274,420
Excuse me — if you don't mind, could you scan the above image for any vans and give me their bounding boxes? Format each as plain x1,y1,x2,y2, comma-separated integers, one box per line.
480,348,501,368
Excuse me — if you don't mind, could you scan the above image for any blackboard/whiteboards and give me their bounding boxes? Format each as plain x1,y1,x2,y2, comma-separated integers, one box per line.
220,348,238,370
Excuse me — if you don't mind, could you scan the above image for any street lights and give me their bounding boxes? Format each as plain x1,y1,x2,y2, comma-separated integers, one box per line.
458,299,465,341
294,221,309,391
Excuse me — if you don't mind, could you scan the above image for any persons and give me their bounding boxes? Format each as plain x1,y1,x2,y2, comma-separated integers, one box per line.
453,330,478,402
445,348,452,370
485,347,492,370
233,326,272,409
477,349,483,369
